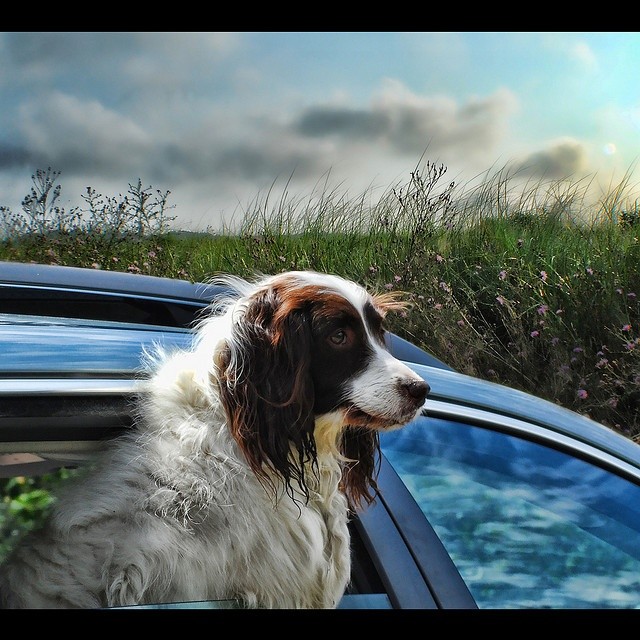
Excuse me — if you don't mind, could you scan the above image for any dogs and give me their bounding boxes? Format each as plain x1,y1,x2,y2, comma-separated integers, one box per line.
1,272,434,608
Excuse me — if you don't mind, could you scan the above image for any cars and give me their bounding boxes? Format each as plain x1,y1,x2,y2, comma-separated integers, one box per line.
0,258,453,371
0,315,640,612
